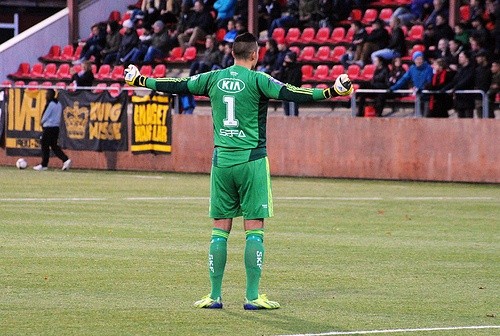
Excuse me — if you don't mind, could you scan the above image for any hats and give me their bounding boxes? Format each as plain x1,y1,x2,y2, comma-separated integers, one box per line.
151,20,165,31
412,51,424,61
471,48,490,57
122,19,133,29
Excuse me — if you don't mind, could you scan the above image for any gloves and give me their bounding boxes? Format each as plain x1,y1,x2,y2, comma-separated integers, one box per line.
333,73,352,96
123,64,142,87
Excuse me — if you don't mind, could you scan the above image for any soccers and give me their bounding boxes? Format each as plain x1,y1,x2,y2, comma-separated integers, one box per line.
15,158,28,170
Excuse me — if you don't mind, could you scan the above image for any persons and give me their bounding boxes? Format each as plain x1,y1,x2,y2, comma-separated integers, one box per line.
32,88,72,171
124,33,352,310
69,0,500,120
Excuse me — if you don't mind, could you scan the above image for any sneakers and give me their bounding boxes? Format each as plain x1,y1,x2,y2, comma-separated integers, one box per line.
193,293,223,309
32,163,47,170
243,295,280,310
61,159,71,171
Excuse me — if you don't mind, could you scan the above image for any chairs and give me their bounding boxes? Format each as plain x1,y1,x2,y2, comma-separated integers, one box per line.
0,0,500,118
